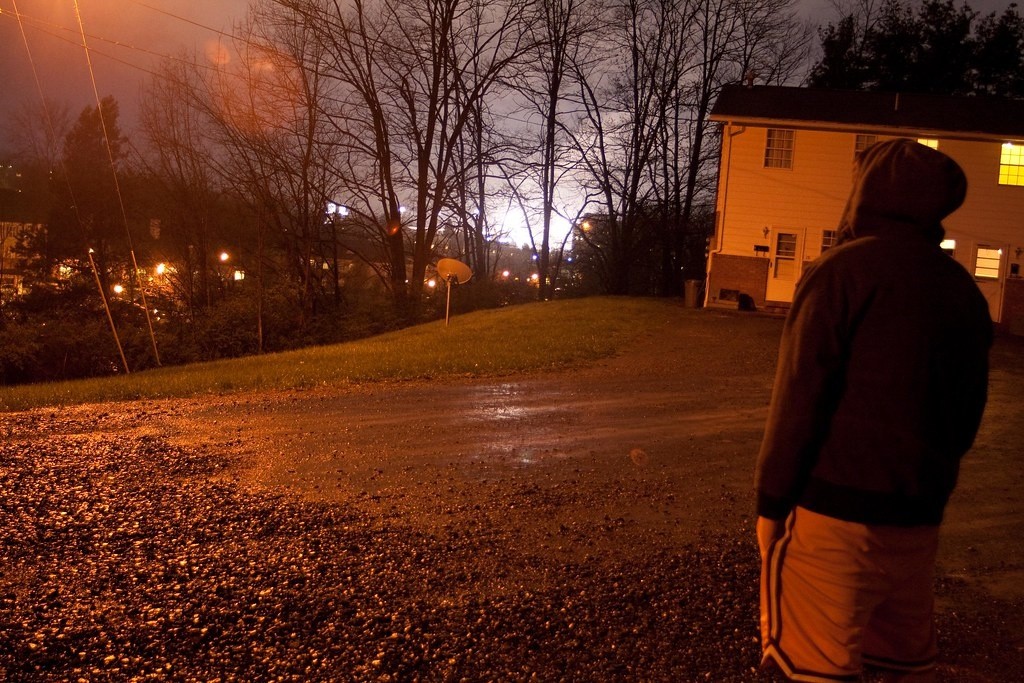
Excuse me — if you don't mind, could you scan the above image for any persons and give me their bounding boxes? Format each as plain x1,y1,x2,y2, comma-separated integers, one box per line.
754,137,993,683
312,270,351,316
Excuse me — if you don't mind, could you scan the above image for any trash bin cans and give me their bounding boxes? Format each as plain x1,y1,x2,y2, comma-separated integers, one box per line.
684,279,702,308
